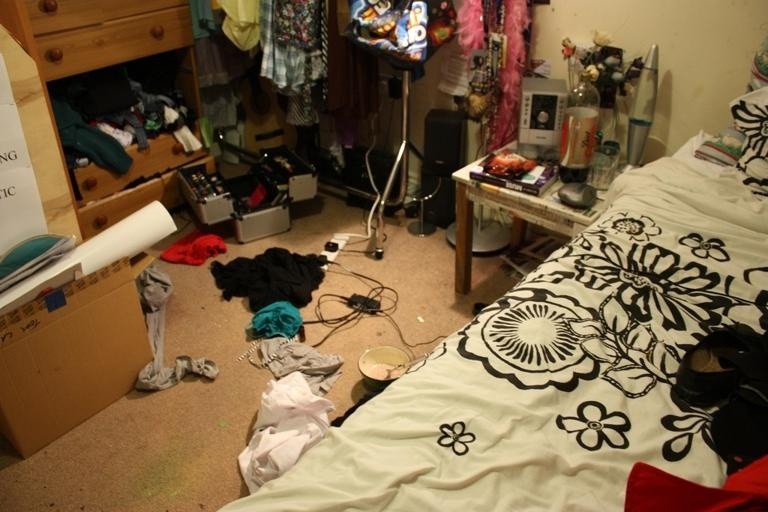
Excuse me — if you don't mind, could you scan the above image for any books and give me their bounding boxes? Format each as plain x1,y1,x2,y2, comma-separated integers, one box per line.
469,152,559,198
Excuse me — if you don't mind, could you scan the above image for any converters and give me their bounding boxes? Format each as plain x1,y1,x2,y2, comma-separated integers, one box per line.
347,292,381,315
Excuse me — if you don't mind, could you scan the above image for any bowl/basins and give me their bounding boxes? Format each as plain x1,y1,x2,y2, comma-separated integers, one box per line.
356,345,413,390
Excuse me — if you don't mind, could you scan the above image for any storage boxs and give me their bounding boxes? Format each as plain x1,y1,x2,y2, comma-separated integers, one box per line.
2,247,164,461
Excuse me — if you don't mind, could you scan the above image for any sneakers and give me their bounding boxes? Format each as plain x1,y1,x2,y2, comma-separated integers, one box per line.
712,380,768,473
673,322,767,404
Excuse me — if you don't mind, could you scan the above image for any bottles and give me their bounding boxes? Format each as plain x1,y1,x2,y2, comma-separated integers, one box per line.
191,172,225,202
557,70,600,183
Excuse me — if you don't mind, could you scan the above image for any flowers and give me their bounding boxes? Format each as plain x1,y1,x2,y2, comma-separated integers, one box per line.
561,26,617,82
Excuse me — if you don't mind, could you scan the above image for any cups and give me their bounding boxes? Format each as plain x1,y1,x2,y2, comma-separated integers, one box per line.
586,141,621,190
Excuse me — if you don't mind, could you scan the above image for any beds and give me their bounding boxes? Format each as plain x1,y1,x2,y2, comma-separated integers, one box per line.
219,129,767,508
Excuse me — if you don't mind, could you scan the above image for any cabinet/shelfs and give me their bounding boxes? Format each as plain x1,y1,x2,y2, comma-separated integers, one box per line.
2,2,216,239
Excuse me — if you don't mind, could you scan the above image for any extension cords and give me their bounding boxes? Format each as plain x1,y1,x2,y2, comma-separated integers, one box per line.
315,232,350,271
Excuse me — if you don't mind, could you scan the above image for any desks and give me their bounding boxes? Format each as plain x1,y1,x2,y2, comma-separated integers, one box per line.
449,128,631,296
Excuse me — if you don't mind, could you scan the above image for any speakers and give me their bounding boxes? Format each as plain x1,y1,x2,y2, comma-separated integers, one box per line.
421,170,455,228
424,109,463,174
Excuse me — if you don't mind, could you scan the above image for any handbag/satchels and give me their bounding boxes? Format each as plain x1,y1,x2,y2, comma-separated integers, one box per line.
346,0,459,65
